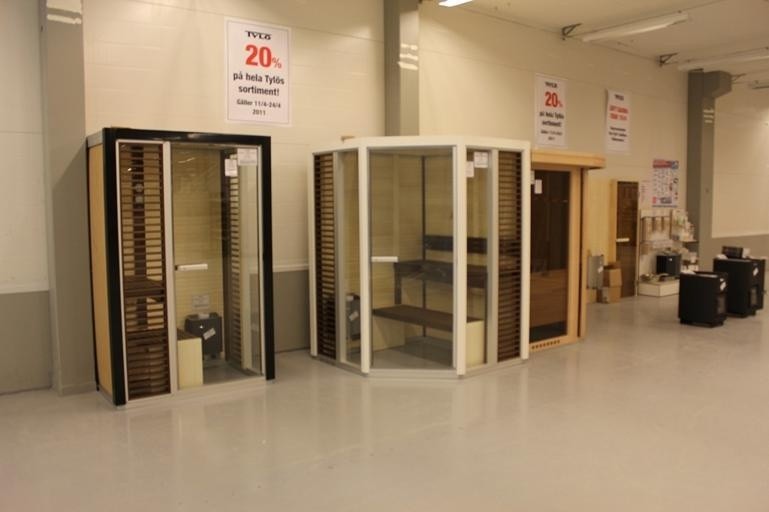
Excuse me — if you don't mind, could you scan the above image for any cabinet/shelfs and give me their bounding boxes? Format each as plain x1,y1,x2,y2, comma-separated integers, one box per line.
637,208,700,298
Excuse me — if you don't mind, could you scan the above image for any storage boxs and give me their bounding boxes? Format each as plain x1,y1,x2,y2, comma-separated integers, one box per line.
596,265,622,303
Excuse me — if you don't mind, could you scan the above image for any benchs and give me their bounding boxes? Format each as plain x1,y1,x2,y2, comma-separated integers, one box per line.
373,304,485,371
